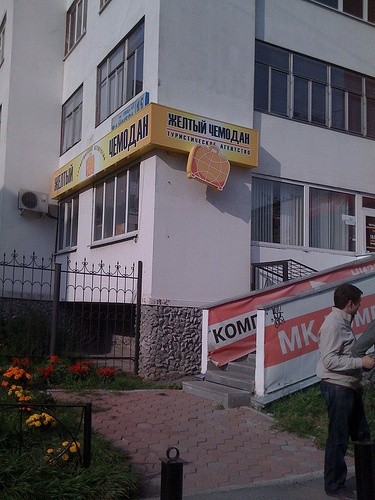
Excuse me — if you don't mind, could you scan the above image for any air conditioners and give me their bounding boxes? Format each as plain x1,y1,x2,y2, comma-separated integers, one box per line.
17,188,48,219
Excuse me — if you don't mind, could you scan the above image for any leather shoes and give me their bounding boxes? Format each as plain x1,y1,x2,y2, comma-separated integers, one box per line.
329,487,354,500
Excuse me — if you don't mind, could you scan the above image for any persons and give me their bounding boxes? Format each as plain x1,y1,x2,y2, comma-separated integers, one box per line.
315,283,375,499
351,318,375,389
